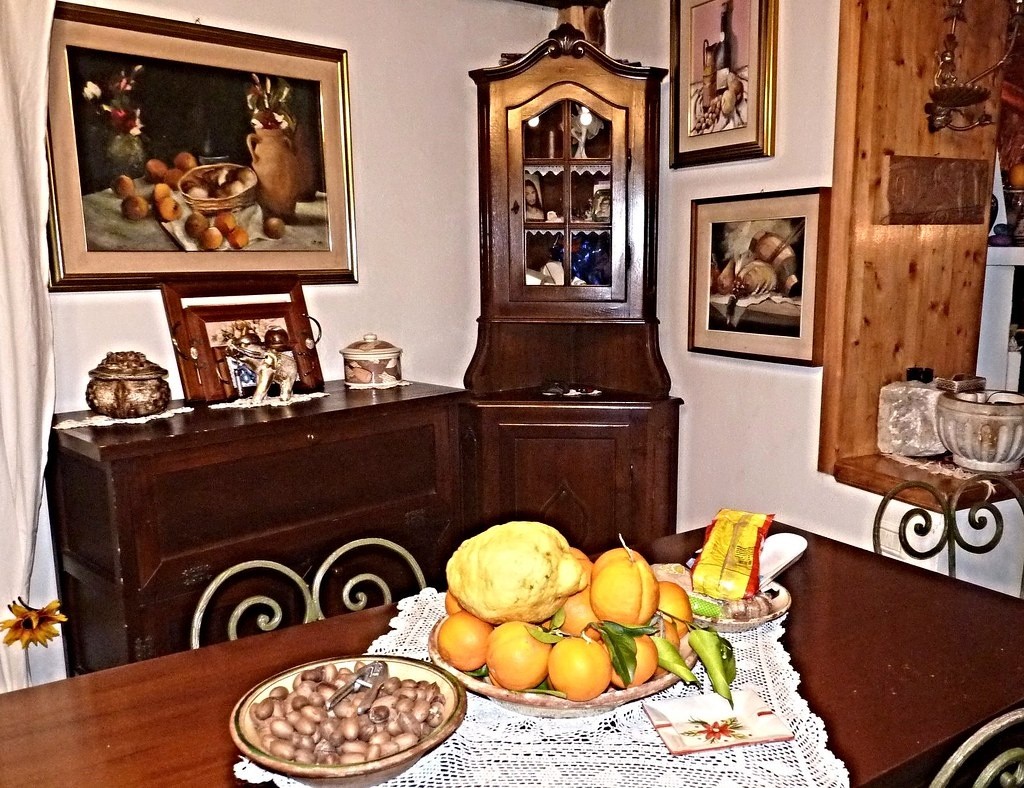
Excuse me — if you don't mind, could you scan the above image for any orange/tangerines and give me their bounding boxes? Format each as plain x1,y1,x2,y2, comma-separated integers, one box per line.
438,546,692,701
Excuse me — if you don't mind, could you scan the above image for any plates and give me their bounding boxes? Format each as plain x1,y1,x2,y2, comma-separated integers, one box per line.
694,579,790,633
642,687,793,754
230,654,467,778
429,615,696,707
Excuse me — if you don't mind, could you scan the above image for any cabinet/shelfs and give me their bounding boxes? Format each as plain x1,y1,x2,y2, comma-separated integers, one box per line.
463,21,684,554
45,380,474,679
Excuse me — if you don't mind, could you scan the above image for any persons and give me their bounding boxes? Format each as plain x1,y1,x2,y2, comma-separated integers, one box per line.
525,180,545,219
597,197,610,217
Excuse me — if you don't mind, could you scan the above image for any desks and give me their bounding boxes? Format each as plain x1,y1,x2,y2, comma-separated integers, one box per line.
0,523,1024,788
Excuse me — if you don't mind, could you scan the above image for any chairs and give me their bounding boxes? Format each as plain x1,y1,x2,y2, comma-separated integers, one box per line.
189,535,427,650
873,472,1024,600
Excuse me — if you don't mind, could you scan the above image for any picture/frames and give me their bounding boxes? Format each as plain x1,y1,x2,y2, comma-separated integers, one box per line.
668,0,779,170
687,187,831,367
45,0,359,294
159,279,326,402
184,301,317,404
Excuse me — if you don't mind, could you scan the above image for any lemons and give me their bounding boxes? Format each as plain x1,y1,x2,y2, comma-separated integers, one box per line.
445,521,589,624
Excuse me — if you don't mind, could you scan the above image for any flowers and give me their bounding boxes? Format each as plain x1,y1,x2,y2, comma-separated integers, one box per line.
0,596,68,650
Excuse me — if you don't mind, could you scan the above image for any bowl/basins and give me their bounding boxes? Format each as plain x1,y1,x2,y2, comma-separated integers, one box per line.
935,389,1024,471
